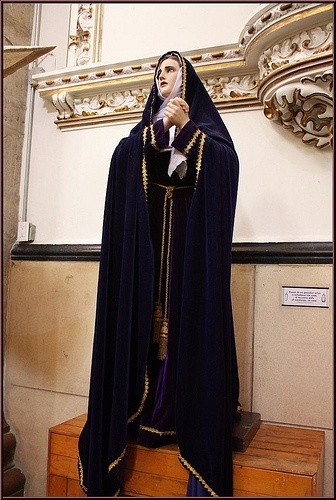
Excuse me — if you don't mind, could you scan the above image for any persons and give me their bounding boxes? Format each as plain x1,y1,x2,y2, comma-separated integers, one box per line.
76,52,241,498
